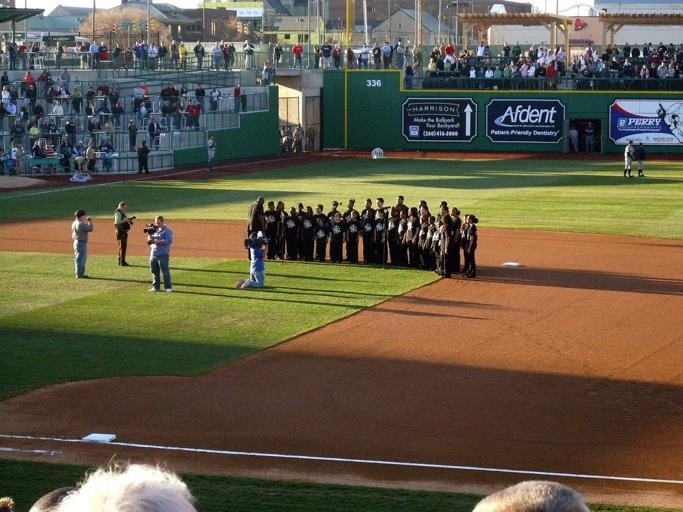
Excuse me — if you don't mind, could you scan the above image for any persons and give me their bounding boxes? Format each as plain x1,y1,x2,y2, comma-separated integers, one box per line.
623,140,645,176
470,479,588,511
266,42,283,69
72,209,95,279
244,194,480,280
146,214,175,293
570,41,682,91
52,456,198,511
371,147,383,159
281,123,315,153
234,230,269,289
568,120,595,153
112,200,134,268
313,38,421,69
292,42,304,68
26,483,79,511
261,61,273,86
1,38,255,176
427,41,566,90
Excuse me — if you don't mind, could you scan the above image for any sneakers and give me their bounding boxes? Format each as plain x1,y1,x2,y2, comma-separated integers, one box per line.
148,287,157,292
265,255,475,279
165,289,171,292
241,280,249,288
236,279,244,288
